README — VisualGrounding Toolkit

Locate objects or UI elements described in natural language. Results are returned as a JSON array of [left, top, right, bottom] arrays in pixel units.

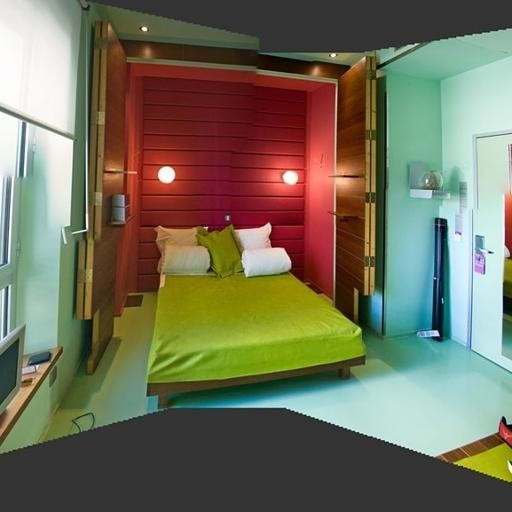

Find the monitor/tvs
[[0, 324, 25, 417]]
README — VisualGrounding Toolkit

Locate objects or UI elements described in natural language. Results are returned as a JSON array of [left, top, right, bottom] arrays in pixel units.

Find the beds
[[146, 254, 367, 409]]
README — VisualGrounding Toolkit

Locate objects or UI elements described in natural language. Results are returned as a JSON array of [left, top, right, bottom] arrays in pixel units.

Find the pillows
[[155, 223, 294, 277]]
